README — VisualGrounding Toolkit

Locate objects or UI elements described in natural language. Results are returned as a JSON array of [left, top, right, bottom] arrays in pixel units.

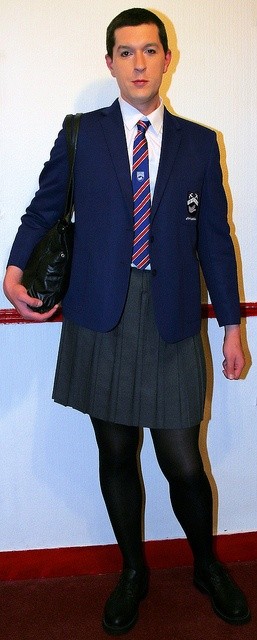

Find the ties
[[132, 120, 151, 271]]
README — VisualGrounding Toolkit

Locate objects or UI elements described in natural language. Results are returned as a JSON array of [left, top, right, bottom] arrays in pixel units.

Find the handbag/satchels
[[22, 211, 70, 314]]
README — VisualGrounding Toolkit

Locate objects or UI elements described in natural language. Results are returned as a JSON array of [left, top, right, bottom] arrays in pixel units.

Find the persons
[[3, 8, 252, 637]]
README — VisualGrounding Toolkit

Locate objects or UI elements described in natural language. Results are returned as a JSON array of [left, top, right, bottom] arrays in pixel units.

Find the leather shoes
[[193, 558, 250, 625], [102, 566, 149, 635]]
